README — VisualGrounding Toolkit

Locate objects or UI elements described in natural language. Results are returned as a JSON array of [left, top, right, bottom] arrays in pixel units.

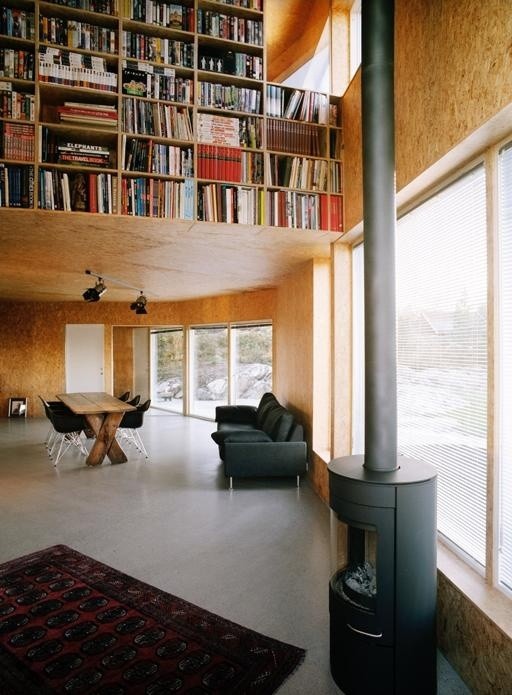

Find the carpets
[[2, 539, 309, 694]]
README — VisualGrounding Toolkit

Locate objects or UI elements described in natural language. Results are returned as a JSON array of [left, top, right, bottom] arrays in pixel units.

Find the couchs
[[214, 392, 310, 492]]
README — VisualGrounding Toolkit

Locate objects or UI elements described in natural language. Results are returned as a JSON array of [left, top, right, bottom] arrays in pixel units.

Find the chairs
[[34, 387, 154, 469]]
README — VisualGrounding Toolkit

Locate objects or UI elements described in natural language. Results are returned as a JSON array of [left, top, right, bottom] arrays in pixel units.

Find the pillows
[[222, 405, 260, 426], [208, 428, 275, 447]]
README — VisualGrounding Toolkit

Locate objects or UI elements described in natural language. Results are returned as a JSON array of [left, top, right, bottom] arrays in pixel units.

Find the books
[[196, 0, 263, 46], [1, 6, 35, 210], [197, 46, 264, 225], [39, 14, 120, 214], [266, 84, 343, 233], [47, 1, 196, 33], [121, 28, 195, 220]]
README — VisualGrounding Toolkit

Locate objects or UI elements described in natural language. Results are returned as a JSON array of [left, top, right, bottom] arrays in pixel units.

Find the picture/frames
[[8, 396, 29, 419]]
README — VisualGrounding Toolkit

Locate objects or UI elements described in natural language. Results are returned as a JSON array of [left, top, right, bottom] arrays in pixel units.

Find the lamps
[[82, 274, 150, 316]]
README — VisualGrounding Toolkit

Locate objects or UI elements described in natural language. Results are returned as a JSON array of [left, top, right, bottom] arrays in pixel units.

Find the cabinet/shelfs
[[0, 1, 346, 234], [325, 471, 440, 694]]
